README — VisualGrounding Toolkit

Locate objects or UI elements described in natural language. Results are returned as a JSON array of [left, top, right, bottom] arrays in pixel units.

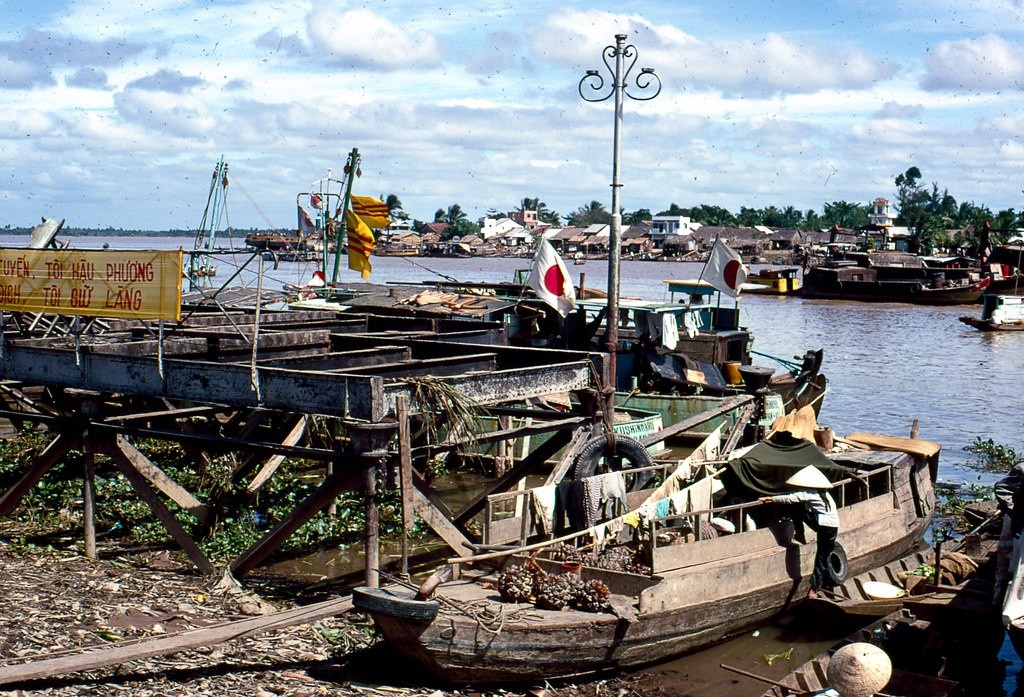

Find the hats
[[786, 464, 833, 489], [827, 641, 892, 697]]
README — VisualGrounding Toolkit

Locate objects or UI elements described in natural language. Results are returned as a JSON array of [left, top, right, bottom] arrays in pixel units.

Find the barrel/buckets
[[1001, 264, 1010, 276], [722, 360, 742, 384]]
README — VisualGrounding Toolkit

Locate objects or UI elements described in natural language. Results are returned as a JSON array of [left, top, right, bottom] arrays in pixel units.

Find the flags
[[526, 235, 577, 320], [310, 192, 328, 210], [347, 191, 392, 229], [701, 233, 749, 296], [297, 204, 317, 234], [341, 210, 376, 282]]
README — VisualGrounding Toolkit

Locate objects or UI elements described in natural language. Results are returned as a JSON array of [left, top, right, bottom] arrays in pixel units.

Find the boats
[[958, 294, 1024, 332], [804, 531, 999, 627], [351, 418, 947, 688], [761, 557, 1007, 697], [262, 251, 322, 262], [744, 243, 991, 306], [283, 269, 829, 474]]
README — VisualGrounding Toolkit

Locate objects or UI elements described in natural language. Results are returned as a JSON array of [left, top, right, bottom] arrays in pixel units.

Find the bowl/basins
[[863, 581, 905, 600]]
